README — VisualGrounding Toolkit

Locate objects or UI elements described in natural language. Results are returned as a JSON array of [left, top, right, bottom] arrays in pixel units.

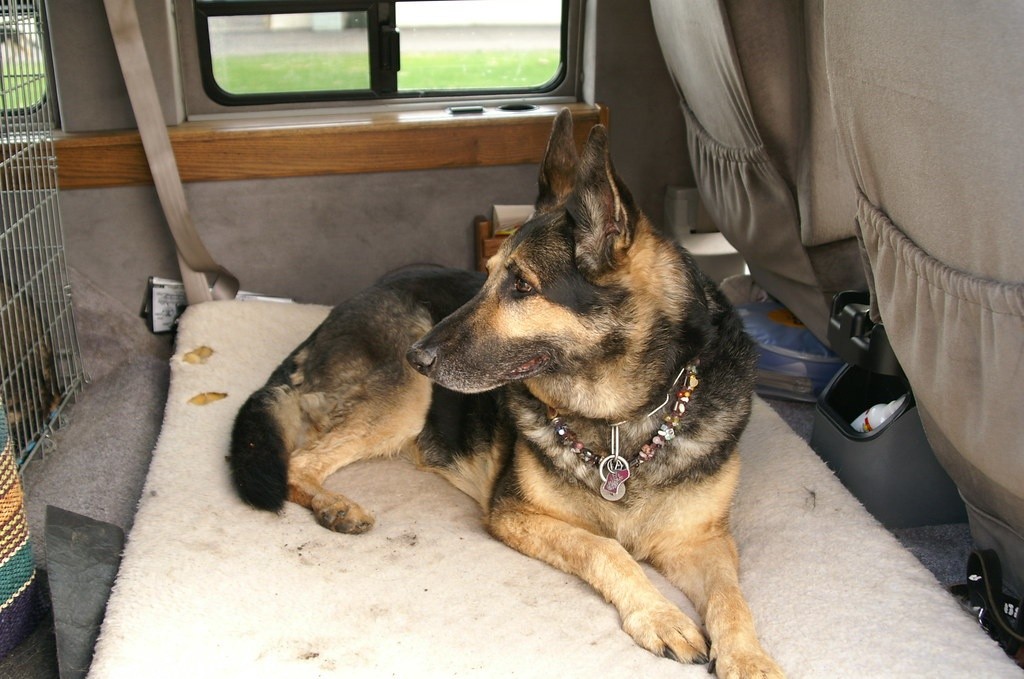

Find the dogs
[[230, 107, 786, 679]]
